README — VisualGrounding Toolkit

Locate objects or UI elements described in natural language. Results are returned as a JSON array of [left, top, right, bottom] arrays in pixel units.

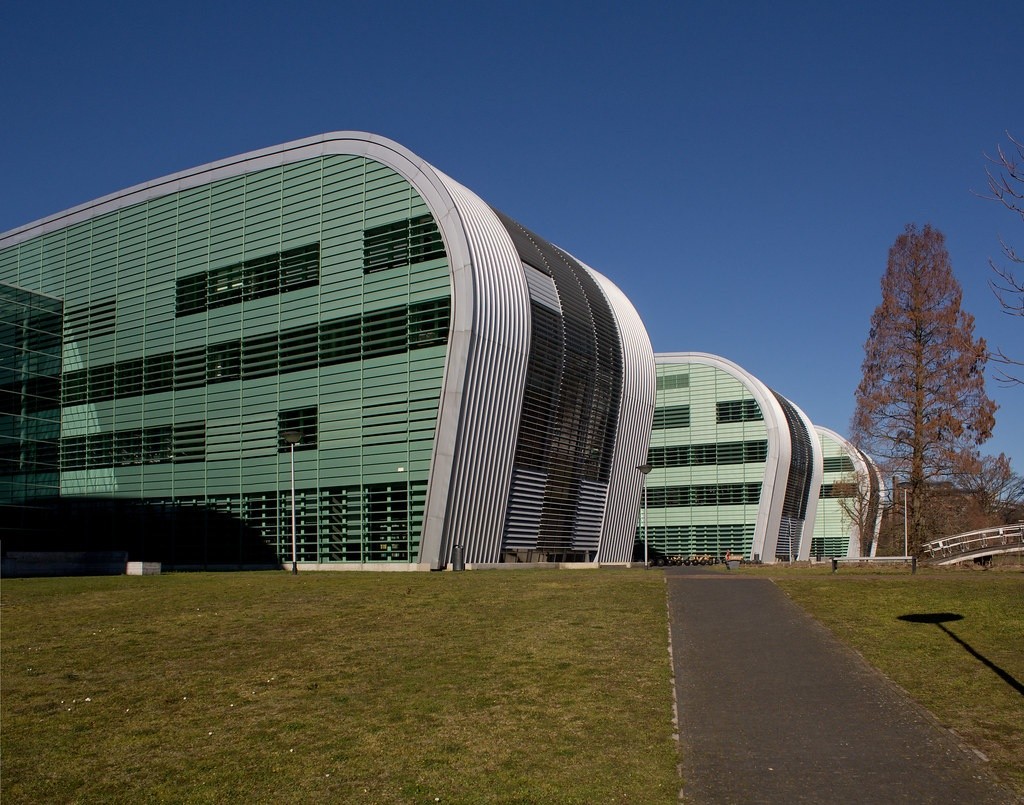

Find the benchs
[[126, 561, 161, 576]]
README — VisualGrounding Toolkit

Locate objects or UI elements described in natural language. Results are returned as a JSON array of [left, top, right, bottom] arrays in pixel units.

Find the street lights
[[636, 465, 655, 566], [787, 511, 795, 565], [280, 429, 306, 574], [897, 482, 912, 557]]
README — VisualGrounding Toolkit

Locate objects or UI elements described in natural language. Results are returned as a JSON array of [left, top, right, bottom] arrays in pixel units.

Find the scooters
[[665, 556, 726, 566]]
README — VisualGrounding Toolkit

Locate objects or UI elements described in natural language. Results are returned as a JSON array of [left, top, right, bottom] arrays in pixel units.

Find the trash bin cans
[[452, 544, 464, 572]]
